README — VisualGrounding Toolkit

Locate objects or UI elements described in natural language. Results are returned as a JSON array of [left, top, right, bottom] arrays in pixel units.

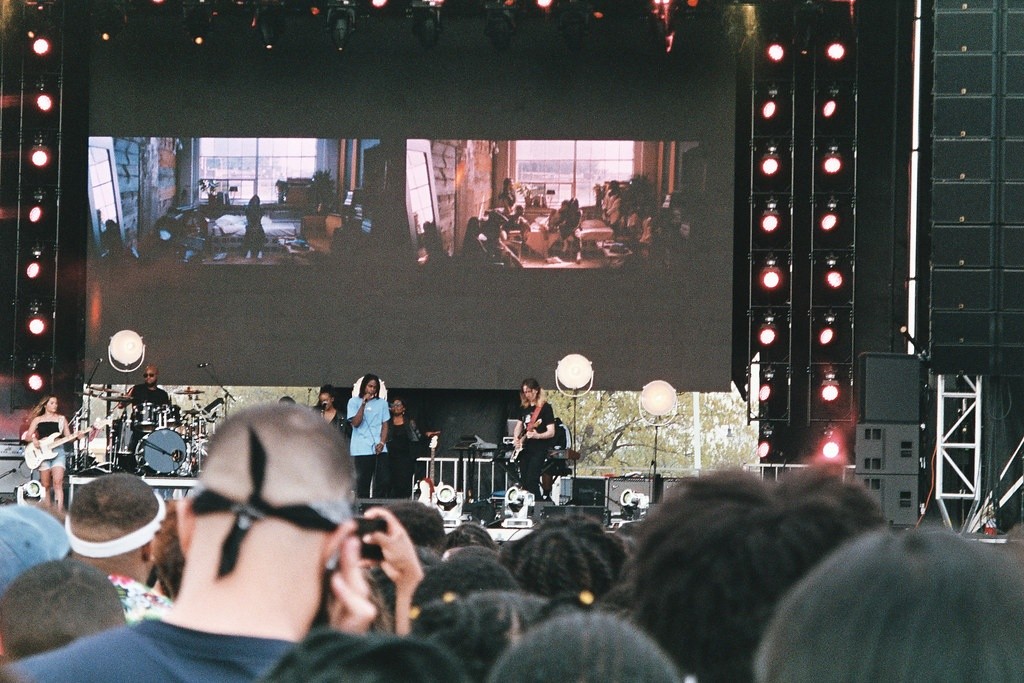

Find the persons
[[242, 195, 266, 258], [315, 384, 352, 439], [752, 529, 1024, 683], [0, 402, 680, 683], [25, 395, 83, 510], [118, 366, 169, 451], [385, 398, 441, 498], [595, 180, 651, 250], [617, 468, 886, 683], [463, 178, 531, 268], [513, 378, 555, 501], [347, 374, 391, 497], [538, 197, 583, 263]]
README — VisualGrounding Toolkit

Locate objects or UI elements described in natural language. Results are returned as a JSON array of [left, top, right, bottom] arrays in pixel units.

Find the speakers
[[607, 476, 693, 519], [0, 459, 41, 492], [540, 504, 607, 528], [853, 350, 921, 527], [572, 476, 607, 506]]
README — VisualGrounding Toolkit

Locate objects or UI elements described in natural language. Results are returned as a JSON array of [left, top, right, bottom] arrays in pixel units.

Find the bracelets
[[381, 442, 384, 445]]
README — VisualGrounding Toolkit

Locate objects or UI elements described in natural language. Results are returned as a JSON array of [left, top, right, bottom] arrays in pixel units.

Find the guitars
[[417, 435, 439, 504], [23, 415, 113, 470], [509, 418, 543, 463]]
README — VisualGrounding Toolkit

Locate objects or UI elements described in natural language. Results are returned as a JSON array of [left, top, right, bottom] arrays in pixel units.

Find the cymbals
[[173, 389, 205, 395], [73, 392, 102, 398], [86, 386, 120, 393], [101, 396, 142, 403]]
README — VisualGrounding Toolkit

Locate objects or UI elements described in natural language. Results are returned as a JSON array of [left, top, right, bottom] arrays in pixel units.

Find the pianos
[[481, 448, 581, 504]]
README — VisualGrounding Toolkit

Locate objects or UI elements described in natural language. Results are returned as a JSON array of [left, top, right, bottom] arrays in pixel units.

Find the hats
[[0, 504, 71, 592]]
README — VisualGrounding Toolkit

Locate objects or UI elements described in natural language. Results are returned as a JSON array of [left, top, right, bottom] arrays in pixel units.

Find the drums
[[132, 400, 157, 426], [134, 426, 187, 477], [150, 405, 179, 422], [106, 419, 141, 456]]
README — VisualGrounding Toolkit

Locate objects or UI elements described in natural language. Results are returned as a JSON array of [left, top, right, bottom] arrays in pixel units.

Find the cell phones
[[353, 517, 386, 560]]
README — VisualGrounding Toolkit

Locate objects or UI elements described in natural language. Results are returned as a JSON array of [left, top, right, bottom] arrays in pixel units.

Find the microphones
[[197, 362, 211, 367], [321, 401, 328, 412], [12, 469, 16, 473], [364, 399, 367, 404]]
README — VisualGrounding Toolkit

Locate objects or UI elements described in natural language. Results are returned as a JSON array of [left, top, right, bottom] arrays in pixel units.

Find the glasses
[[143, 372, 157, 378]]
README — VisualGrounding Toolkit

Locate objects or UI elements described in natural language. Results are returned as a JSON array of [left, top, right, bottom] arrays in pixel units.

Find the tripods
[[76, 408, 120, 477]]
